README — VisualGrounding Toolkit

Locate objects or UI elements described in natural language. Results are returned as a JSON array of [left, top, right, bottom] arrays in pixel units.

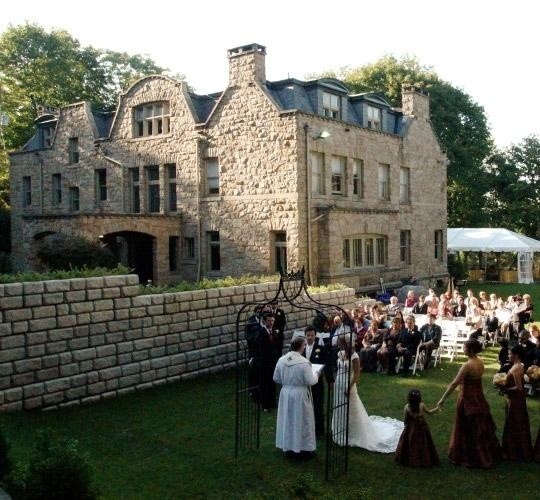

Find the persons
[[248, 313, 284, 413], [394, 389, 444, 468], [436, 338, 503, 470], [243, 288, 540, 377], [272, 336, 319, 462], [494, 345, 534, 463], [300, 326, 334, 439], [331, 333, 405, 454]]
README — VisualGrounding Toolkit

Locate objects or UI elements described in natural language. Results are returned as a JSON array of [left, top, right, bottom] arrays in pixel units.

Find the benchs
[[387, 314, 498, 376]]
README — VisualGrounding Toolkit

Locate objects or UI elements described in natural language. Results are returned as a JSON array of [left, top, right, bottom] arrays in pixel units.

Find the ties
[[269, 330, 272, 342]]
